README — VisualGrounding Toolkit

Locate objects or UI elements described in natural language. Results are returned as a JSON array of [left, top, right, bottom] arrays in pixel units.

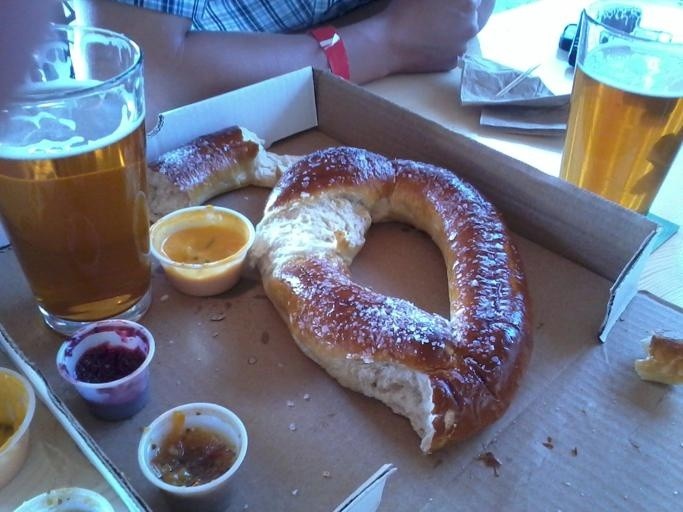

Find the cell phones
[[559, 24, 674, 53]]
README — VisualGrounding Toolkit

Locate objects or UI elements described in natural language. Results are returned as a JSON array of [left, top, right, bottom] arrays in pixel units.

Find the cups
[[0, 23, 152, 339], [0, 367, 36, 491], [150, 205, 256, 297], [14, 488, 115, 512], [138, 402, 247, 502], [57, 320, 156, 418], [556, 1, 682, 222]]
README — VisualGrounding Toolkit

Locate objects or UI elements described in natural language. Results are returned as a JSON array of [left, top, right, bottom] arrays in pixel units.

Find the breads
[[148, 124, 305, 223], [256, 147, 533, 455], [635, 329, 683, 385]]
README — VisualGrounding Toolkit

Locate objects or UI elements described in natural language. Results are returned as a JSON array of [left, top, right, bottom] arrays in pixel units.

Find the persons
[[1, 0, 495, 135]]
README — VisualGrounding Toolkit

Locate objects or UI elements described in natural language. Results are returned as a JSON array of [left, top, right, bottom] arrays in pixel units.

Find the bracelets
[[307, 24, 351, 81]]
